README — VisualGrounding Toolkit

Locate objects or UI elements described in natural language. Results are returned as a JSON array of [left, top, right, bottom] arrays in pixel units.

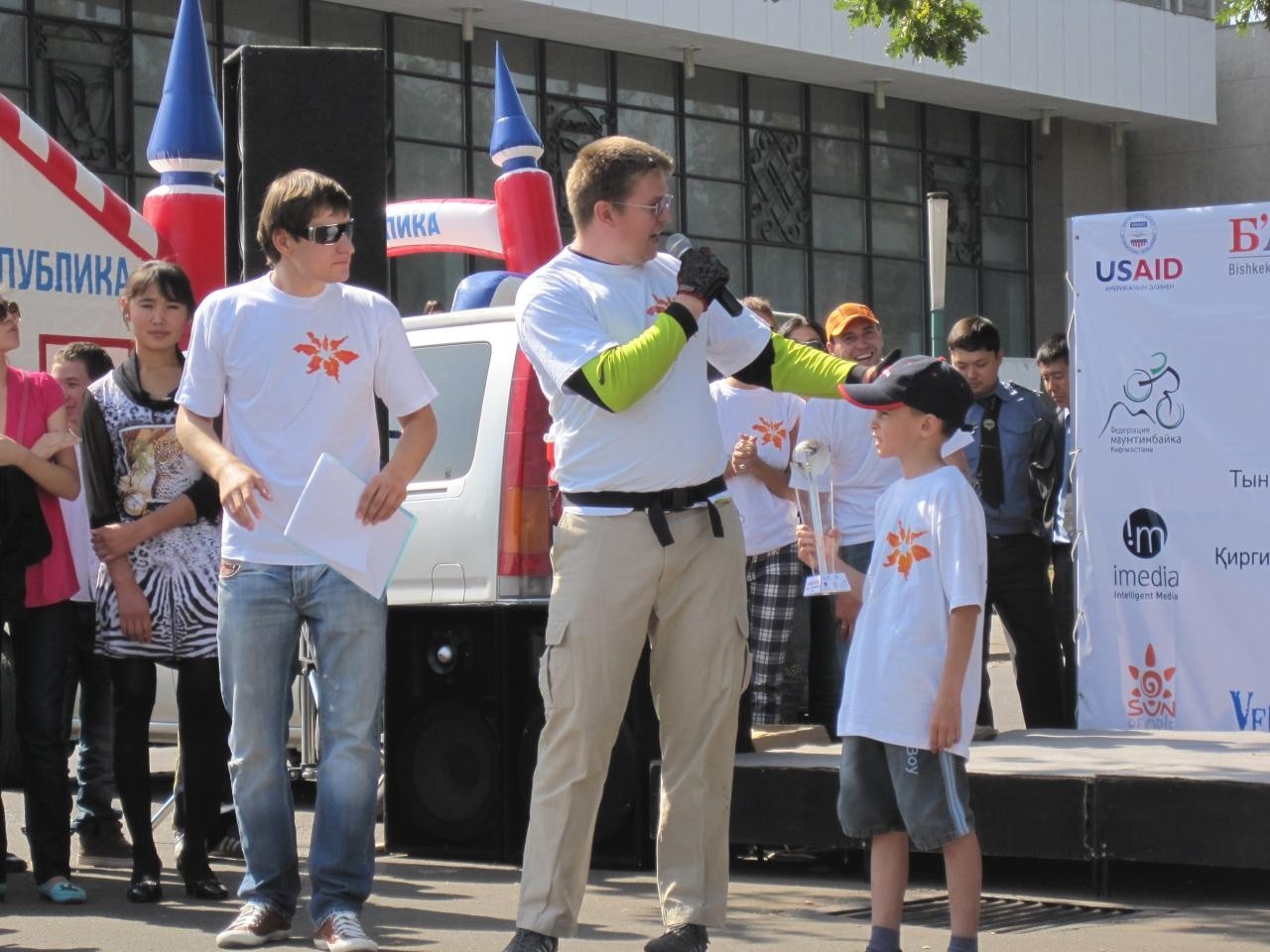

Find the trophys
[[793, 439, 852, 598]]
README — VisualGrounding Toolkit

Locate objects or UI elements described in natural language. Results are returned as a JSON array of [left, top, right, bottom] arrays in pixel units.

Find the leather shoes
[[35, 877, 88, 904]]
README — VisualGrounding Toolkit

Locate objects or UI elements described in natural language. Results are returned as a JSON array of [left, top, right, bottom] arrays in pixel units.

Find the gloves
[[675, 244, 730, 313], [867, 348, 902, 385]]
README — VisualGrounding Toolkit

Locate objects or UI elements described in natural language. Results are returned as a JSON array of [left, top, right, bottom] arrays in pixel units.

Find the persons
[[800, 357, 987, 952], [2, 261, 244, 904], [175, 170, 437, 951], [710, 299, 811, 727], [504, 137, 902, 952], [790, 304, 974, 717], [947, 314, 1077, 732], [779, 316, 829, 357]]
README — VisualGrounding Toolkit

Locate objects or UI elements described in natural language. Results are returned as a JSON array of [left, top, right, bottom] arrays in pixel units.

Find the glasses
[[0, 302, 20, 322], [294, 217, 355, 247], [613, 193, 675, 218], [794, 337, 826, 354]]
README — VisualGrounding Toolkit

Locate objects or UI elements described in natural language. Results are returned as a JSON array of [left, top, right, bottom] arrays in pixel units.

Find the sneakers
[[170, 826, 244, 860], [77, 836, 135, 868], [216, 900, 294, 947], [313, 910, 378, 952], [501, 928, 560, 952], [643, 923, 712, 952]]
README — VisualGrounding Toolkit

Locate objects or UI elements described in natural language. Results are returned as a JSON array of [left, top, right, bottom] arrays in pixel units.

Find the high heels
[[174, 851, 229, 900], [126, 857, 161, 904]]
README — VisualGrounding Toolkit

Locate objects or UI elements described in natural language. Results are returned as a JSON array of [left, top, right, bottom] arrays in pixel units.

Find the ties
[[976, 393, 1009, 512]]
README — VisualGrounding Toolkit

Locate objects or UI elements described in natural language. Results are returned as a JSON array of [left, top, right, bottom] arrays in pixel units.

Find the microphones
[[666, 233, 743, 317]]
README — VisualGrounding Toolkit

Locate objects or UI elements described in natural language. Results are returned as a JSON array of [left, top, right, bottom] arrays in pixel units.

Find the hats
[[824, 302, 880, 344], [837, 353, 972, 433]]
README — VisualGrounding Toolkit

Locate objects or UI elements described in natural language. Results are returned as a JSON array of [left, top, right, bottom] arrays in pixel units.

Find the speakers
[[385, 598, 660, 870], [218, 46, 391, 297]]
